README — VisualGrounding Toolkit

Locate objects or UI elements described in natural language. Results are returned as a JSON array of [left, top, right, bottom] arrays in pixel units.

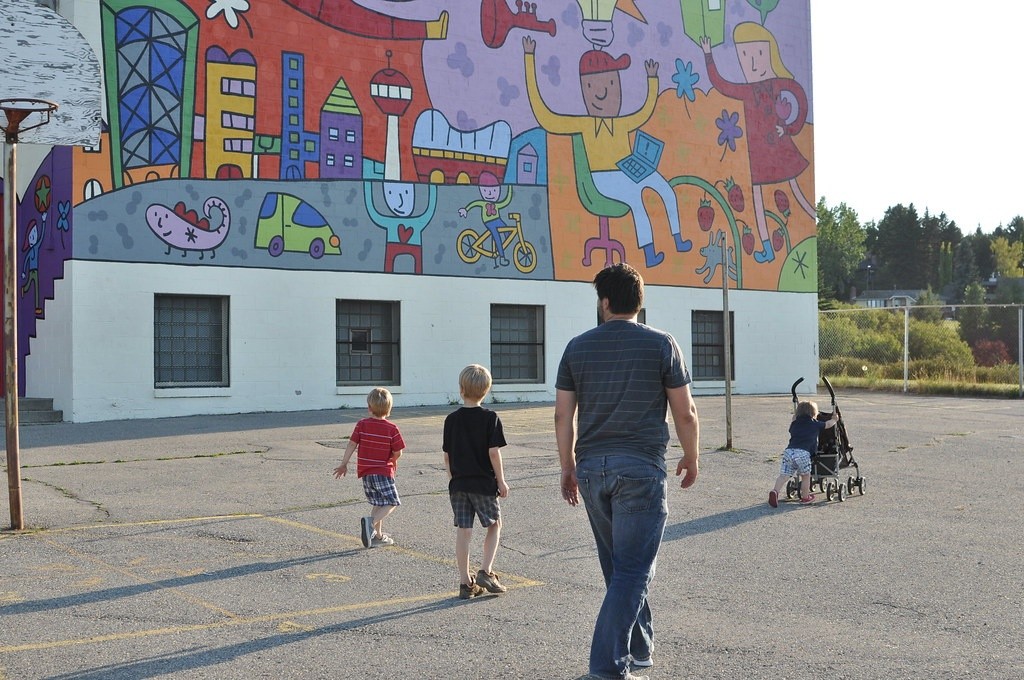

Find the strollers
[[785, 373, 867, 504]]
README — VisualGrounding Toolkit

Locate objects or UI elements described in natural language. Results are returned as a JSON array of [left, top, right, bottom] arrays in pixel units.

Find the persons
[[768, 401, 839, 508], [332, 388, 405, 549], [442, 364, 509, 600], [554, 263, 700, 680]]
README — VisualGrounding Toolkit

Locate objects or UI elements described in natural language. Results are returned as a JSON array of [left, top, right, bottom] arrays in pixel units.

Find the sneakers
[[459, 575, 486, 598], [371, 534, 393, 547], [476, 570, 506, 593], [361, 517, 377, 548]]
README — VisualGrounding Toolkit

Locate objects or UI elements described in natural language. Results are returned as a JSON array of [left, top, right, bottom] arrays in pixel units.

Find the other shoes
[[800, 495, 816, 504], [632, 656, 653, 666], [627, 673, 649, 680], [768, 489, 778, 507]]
